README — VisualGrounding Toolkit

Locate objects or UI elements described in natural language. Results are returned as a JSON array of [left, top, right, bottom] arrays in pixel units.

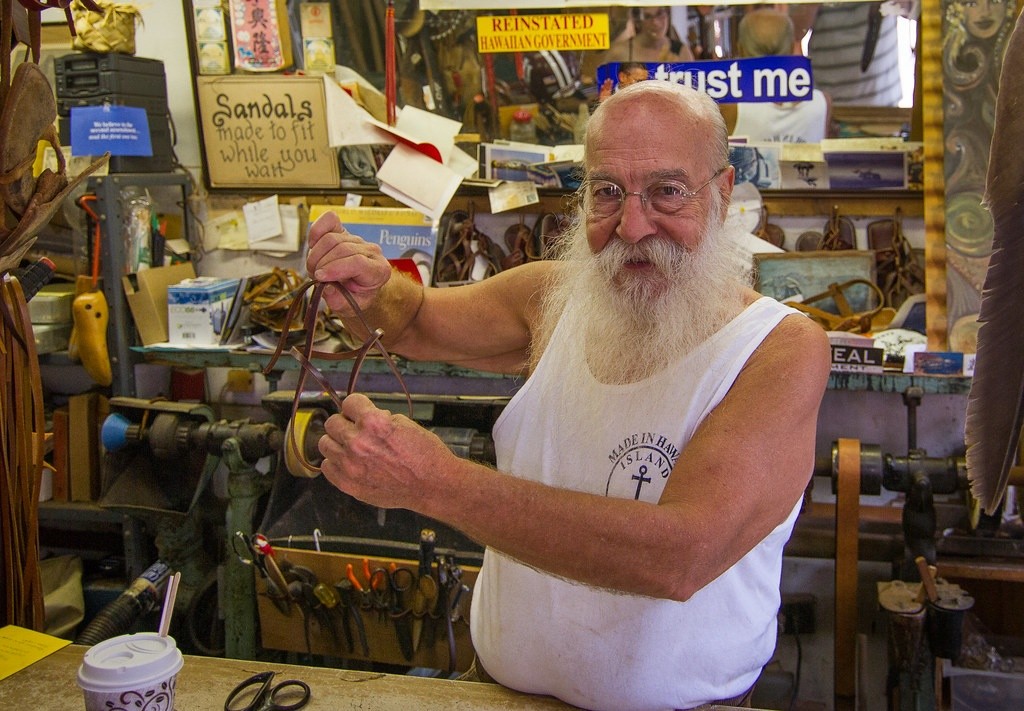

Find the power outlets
[[783, 596, 816, 633]]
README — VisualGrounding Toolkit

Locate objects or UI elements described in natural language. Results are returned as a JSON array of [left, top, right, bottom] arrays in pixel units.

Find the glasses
[[578, 171, 721, 217], [643, 9, 667, 25]]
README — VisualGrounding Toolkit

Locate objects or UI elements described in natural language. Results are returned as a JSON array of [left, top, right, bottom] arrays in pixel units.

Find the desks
[[0, 643, 750, 710]]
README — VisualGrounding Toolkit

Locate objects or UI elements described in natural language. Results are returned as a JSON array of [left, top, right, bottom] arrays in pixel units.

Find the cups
[[77, 632, 184, 711]]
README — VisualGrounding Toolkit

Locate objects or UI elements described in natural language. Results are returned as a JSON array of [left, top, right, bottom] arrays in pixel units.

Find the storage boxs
[[166, 276, 247, 346], [26, 283, 77, 355], [53, 47, 174, 173], [121, 263, 196, 344]]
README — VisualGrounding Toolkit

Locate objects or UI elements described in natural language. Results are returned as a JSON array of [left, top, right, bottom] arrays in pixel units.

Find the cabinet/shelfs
[[17, 168, 198, 635]]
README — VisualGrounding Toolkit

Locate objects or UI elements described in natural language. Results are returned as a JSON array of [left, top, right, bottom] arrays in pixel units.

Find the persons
[[720, 11, 830, 142], [587, 7, 696, 80], [306, 79, 830, 711]]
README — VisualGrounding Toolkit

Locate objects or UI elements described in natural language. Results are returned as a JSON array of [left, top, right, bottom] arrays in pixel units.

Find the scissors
[[411, 528, 449, 623], [224, 670, 311, 711], [369, 568, 414, 664]]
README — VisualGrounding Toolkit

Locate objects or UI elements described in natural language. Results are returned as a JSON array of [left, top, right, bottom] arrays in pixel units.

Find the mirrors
[[179, 0, 924, 200]]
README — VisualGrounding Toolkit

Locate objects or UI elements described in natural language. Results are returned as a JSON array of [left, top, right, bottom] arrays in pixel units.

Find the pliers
[[233, 532, 397, 659], [418, 558, 470, 672]]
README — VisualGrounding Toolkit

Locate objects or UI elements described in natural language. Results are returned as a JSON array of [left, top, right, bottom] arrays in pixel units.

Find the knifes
[[413, 529, 437, 653]]
